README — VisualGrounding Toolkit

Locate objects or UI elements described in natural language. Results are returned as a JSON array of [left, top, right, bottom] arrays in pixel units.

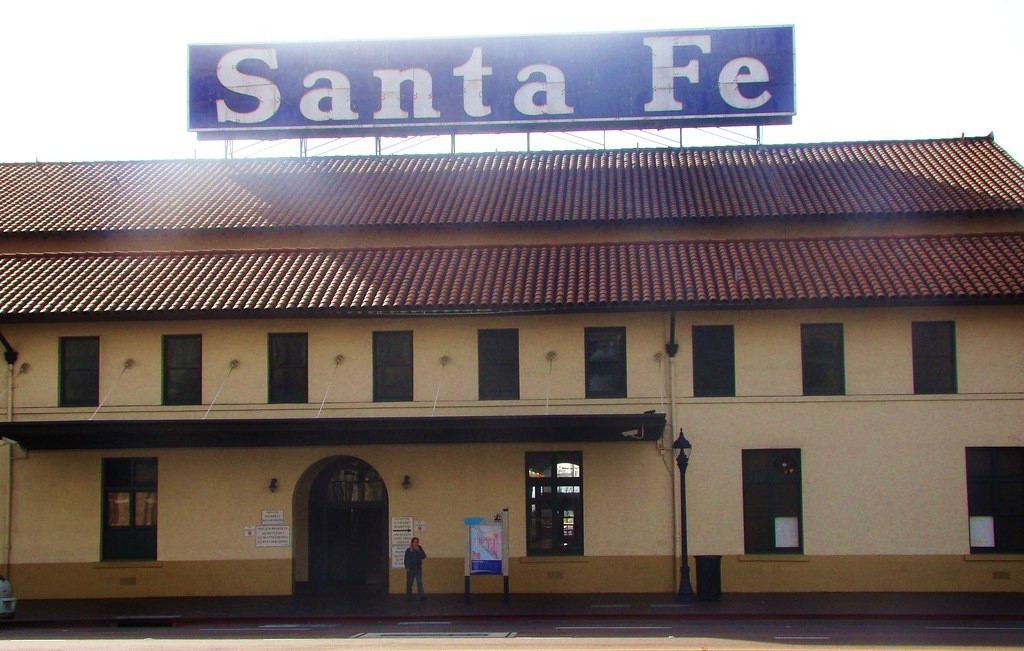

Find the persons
[[403, 537, 429, 601]]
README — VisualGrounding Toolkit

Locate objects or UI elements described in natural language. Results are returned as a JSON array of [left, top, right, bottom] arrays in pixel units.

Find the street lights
[[672, 427, 697, 606]]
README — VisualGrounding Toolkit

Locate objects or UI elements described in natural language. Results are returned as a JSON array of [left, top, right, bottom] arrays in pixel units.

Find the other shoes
[[407, 598, 412, 601], [420, 596, 427, 601]]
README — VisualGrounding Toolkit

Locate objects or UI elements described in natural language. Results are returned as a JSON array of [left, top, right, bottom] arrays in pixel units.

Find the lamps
[[402, 475, 411, 490], [268, 478, 278, 492]]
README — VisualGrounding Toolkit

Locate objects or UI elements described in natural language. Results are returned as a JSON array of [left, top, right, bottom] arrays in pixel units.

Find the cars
[[0, 573, 18, 622]]
